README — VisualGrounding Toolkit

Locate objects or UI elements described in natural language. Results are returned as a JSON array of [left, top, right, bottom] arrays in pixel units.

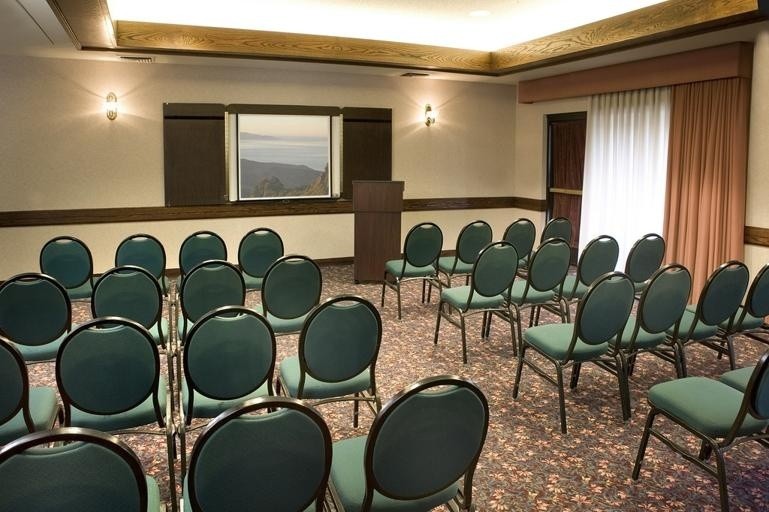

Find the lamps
[[107, 93, 117, 119], [426, 103, 434, 126]]
